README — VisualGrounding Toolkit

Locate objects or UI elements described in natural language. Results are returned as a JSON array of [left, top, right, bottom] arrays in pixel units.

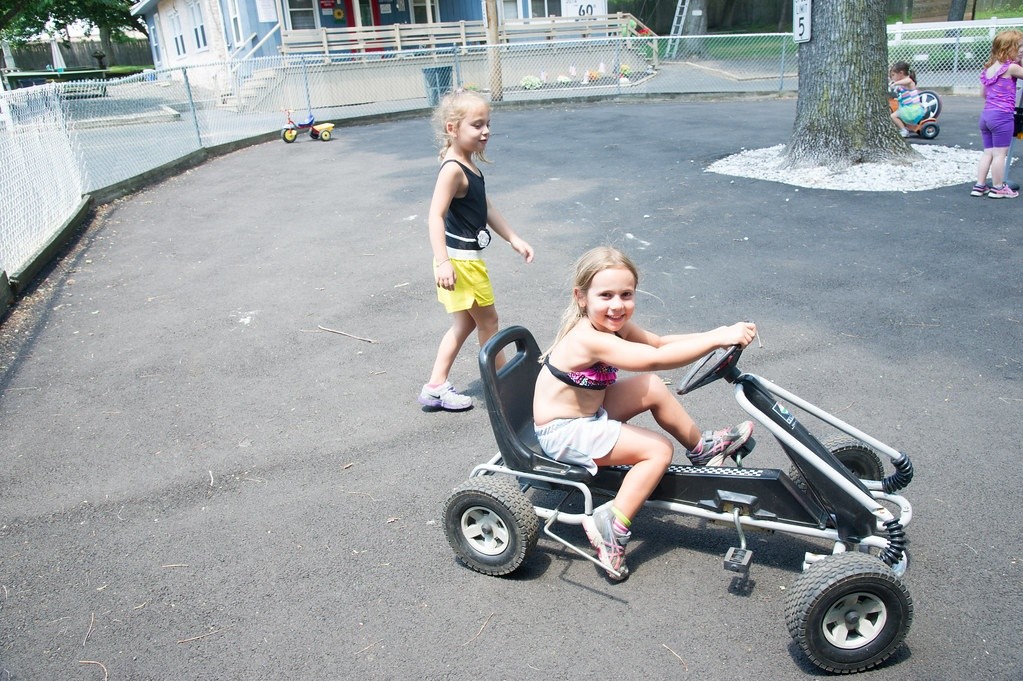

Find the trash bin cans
[[422, 65, 453, 107], [143, 69, 155, 81]]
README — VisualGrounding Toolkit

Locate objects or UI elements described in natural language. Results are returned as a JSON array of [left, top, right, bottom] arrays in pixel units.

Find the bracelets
[[437, 258, 450, 267]]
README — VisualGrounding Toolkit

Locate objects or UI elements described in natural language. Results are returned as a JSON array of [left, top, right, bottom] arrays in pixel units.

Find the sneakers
[[419, 378, 473, 409], [581, 508, 631, 580], [685, 421, 753, 467], [970, 184, 991, 196], [899, 130, 910, 138], [988, 182, 1019, 198]]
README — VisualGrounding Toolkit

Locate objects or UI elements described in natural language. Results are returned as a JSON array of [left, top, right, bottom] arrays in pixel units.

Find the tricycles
[[885, 83, 939, 140], [282, 108, 334, 143]]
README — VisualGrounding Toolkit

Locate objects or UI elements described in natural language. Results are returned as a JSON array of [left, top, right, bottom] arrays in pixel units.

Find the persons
[[889, 62, 925, 138], [532, 245, 756, 581], [970, 30, 1023, 198], [418, 86, 534, 411]]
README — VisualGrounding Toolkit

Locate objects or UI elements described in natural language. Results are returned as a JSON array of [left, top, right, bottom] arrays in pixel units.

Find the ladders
[[665, 0, 690, 60]]
